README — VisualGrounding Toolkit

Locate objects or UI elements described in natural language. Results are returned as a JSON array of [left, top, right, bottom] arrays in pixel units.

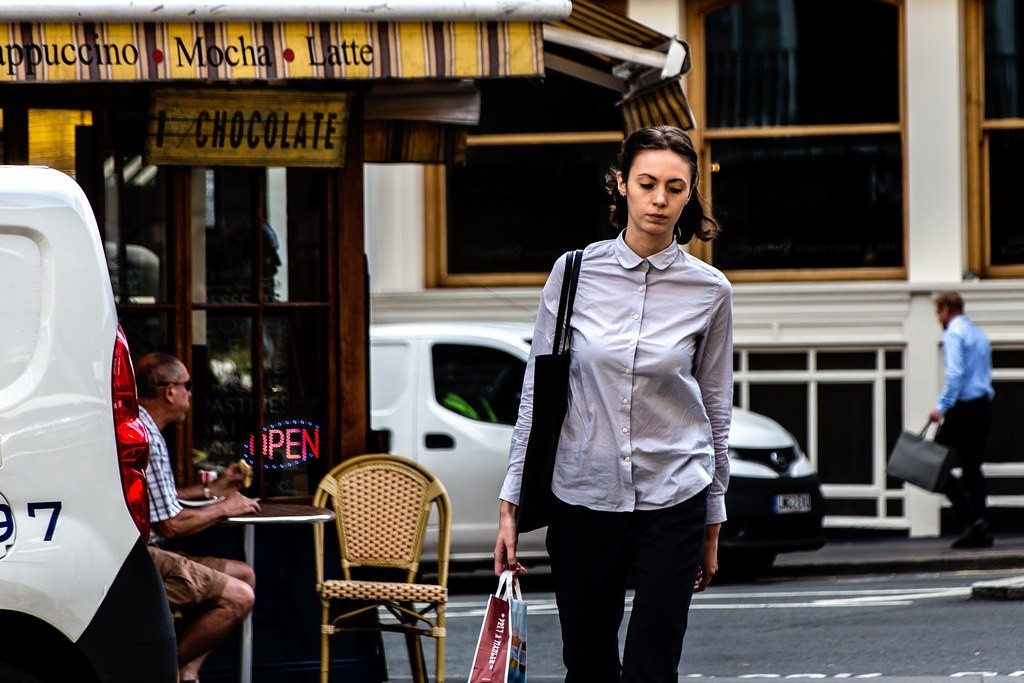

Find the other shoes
[[952, 517, 986, 550], [962, 535, 994, 550]]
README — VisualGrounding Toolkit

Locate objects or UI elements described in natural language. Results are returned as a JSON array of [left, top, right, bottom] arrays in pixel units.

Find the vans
[[368, 320, 828, 588]]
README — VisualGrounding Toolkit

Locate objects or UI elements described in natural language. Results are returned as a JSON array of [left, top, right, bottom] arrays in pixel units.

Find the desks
[[219, 504, 335, 683]]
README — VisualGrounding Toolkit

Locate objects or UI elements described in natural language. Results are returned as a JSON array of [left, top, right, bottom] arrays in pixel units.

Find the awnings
[[0, 0, 699, 165]]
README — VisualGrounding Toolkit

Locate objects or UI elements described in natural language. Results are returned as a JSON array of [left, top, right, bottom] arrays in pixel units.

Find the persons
[[928, 291, 1000, 549], [133, 351, 255, 683], [496, 124, 734, 683], [442, 349, 495, 424]]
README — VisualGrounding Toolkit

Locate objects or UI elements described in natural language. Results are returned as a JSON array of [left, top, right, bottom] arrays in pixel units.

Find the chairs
[[311, 453, 452, 683]]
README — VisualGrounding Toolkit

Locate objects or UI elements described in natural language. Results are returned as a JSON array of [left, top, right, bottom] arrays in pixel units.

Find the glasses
[[157, 377, 191, 391]]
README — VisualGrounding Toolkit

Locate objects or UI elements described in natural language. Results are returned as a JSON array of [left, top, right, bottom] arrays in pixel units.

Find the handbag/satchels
[[467, 571, 528, 683], [513, 250, 584, 534], [885, 418, 951, 492]]
